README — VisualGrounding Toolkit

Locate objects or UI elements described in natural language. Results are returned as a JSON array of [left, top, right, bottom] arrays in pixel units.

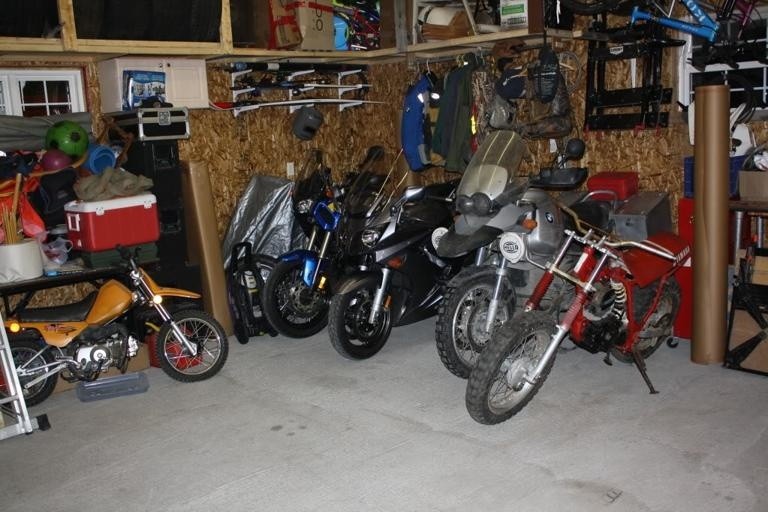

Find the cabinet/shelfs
[[0, 0, 609, 115]]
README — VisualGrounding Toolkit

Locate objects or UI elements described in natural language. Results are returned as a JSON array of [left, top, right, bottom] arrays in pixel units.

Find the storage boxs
[[64, 191, 161, 252], [571, 154, 751, 349]]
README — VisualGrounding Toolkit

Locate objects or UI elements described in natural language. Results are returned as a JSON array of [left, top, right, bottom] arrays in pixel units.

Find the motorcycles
[[0, 244, 230, 410]]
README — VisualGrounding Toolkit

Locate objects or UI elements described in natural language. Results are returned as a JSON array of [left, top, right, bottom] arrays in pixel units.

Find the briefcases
[[614, 191, 672, 240], [105, 106, 191, 142], [587, 171, 639, 201]]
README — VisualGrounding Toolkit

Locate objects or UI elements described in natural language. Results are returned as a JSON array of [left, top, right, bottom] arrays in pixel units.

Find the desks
[[0, 259, 162, 318]]
